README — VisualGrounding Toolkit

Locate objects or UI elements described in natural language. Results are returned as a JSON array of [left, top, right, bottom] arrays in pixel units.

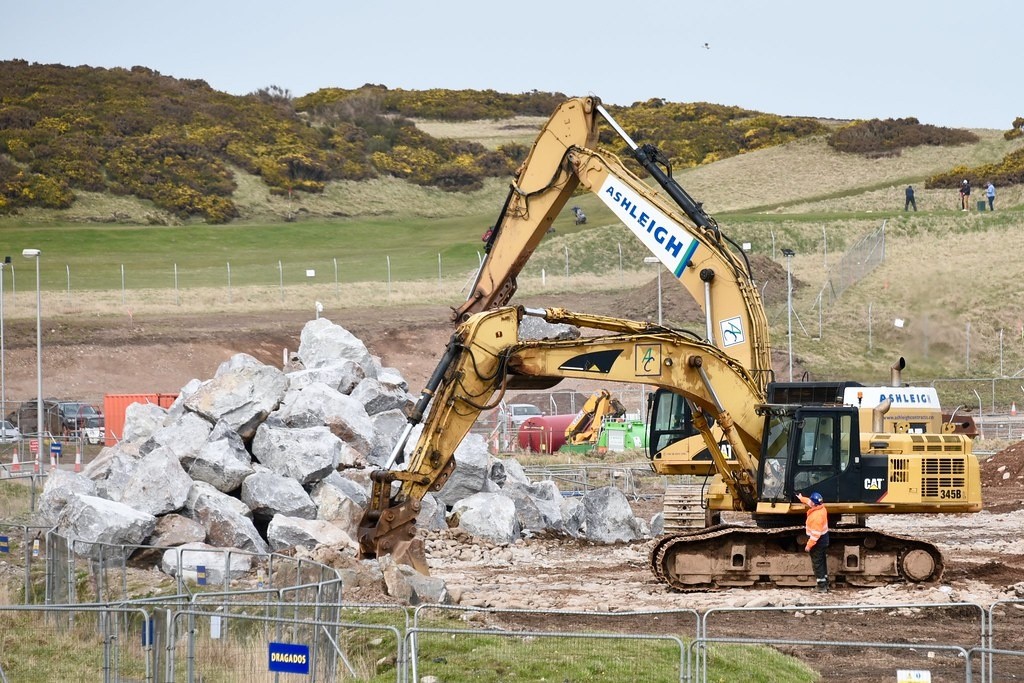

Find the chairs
[[814, 434, 833, 464]]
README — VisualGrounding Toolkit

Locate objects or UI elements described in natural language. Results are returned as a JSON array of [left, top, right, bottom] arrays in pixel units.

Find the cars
[[0, 420, 22, 444], [47, 402, 105, 436]]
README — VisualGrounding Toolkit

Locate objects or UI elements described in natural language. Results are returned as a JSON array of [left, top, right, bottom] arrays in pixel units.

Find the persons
[[796, 493, 831, 588], [905, 186, 917, 212], [960, 180, 970, 212], [982, 180, 995, 211]]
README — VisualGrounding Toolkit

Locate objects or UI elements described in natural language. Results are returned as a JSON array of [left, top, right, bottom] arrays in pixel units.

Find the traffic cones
[[33, 450, 39, 474], [10, 449, 21, 473], [1009, 401, 1016, 416], [50, 453, 57, 469], [73, 448, 81, 473]]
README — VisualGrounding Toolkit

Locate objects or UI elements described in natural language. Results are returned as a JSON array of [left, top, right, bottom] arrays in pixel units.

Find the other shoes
[[811, 588, 828, 593]]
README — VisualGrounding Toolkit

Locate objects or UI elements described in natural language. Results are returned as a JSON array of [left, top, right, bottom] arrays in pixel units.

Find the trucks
[[497, 403, 546, 433]]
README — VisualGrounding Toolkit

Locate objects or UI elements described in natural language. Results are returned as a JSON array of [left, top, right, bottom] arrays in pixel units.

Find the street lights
[[780, 248, 794, 383], [21, 248, 45, 483]]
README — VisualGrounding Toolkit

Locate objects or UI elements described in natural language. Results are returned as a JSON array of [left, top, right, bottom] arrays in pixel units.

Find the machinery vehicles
[[557, 387, 649, 453], [354, 93, 983, 593]]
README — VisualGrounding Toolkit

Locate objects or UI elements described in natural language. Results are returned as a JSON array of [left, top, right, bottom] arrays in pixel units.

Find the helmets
[[810, 492, 823, 505]]
